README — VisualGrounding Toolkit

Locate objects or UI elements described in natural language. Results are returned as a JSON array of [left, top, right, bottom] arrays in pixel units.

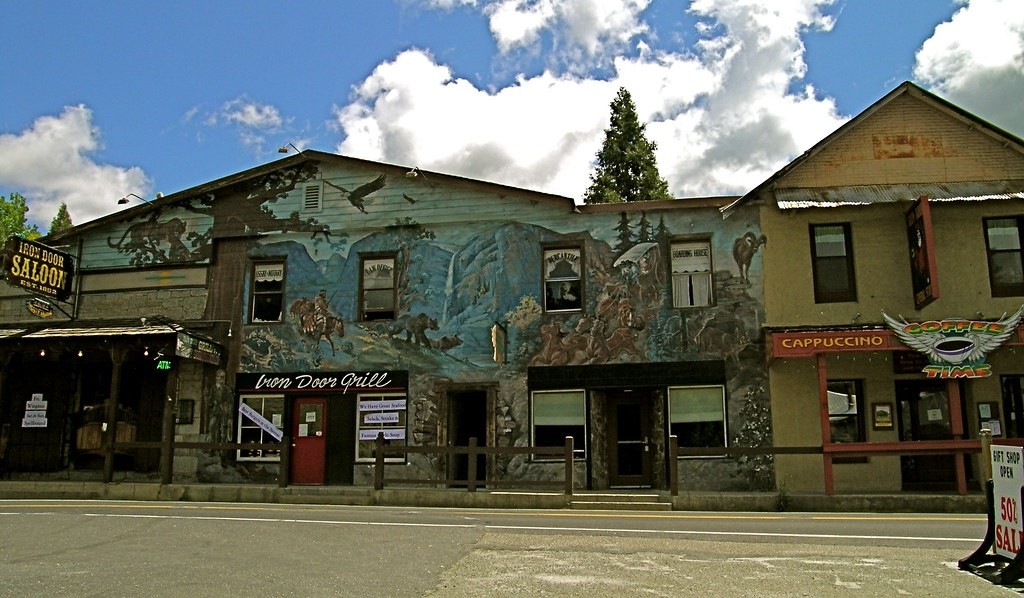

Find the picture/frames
[[872, 402, 894, 432]]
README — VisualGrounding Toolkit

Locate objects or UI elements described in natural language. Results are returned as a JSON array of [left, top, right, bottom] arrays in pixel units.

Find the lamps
[[405, 166, 433, 189], [278, 142, 306, 159], [118, 194, 153, 207]]
